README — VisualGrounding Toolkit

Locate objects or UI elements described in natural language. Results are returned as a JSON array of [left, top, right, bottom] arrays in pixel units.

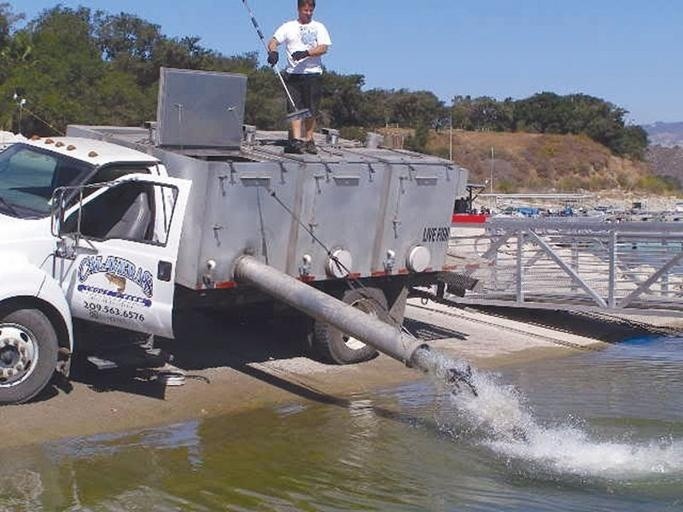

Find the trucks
[[0, 66, 464, 409]]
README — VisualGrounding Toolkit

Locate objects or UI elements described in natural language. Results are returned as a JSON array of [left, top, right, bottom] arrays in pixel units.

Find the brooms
[[240, 0, 312, 122]]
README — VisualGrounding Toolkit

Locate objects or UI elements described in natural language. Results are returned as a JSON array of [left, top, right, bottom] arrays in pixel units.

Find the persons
[[454, 196, 489, 217], [266, 1, 333, 155]]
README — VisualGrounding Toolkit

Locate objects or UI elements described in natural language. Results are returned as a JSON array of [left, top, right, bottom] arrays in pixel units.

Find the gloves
[[291, 50, 310, 61], [267, 51, 278, 68]]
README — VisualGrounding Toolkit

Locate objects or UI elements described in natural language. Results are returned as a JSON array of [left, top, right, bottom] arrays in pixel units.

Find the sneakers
[[305, 138, 317, 155], [284, 138, 303, 153]]
